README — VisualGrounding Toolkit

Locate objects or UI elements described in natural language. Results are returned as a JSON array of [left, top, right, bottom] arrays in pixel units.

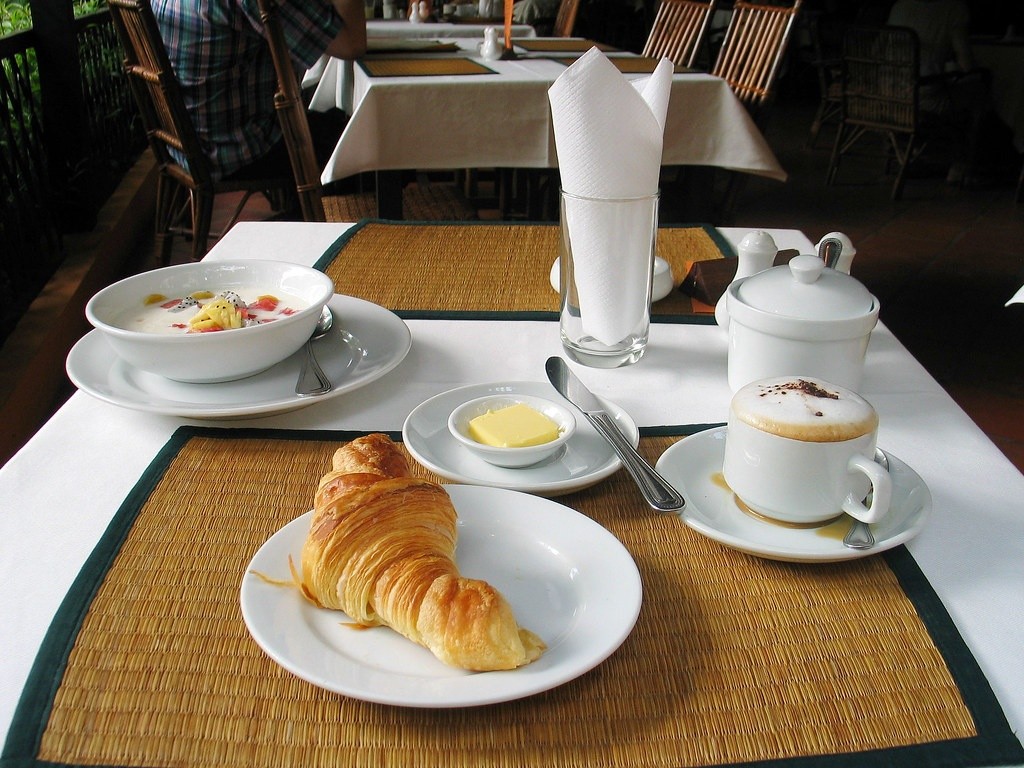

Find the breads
[[288, 431, 549, 671]]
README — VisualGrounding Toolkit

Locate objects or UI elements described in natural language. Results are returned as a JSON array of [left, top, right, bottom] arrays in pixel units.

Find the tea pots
[[479, 0, 492, 19]]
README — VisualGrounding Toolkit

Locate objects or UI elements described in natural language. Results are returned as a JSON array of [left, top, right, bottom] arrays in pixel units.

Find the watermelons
[[200, 298, 300, 331]]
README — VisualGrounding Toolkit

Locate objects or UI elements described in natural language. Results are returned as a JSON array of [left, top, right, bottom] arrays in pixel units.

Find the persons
[[871, 0, 1013, 178], [150, 0, 367, 221]]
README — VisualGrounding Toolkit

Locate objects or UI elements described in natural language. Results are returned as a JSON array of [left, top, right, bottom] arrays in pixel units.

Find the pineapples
[[191, 300, 243, 331]]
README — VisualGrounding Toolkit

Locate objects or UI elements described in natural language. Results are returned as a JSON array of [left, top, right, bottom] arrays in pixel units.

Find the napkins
[[548, 45, 675, 344]]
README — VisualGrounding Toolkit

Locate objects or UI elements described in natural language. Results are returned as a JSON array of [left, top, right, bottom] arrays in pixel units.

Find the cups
[[456, 6, 467, 17], [365, 0, 375, 20], [443, 4, 452, 14], [559, 184, 661, 370], [721, 403, 892, 523], [383, 5, 394, 20], [726, 254, 881, 395]]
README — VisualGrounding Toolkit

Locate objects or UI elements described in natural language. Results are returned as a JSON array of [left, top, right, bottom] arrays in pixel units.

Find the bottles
[[715, 230, 778, 333], [409, 2, 429, 24], [813, 232, 858, 275], [479, 28, 503, 60]]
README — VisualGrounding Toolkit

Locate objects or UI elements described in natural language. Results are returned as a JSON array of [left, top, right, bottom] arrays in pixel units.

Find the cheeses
[[468, 402, 559, 448]]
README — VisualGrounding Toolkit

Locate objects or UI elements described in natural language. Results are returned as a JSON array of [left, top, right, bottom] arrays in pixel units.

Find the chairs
[[644, 0, 714, 73], [111, 1, 295, 263], [805, 15, 912, 156], [551, 0, 577, 38], [715, 0, 802, 108], [254, 0, 477, 221], [822, 26, 978, 202]]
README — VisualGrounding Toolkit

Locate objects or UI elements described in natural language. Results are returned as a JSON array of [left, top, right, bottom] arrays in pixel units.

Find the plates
[[240, 484, 642, 708], [64, 293, 413, 421], [655, 426, 932, 563], [403, 381, 640, 498]]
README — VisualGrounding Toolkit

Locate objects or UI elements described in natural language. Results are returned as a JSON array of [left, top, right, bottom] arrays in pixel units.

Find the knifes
[[545, 356, 688, 515]]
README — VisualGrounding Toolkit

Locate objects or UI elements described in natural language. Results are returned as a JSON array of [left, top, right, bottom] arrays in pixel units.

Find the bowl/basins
[[85, 259, 335, 385], [446, 394, 577, 469]]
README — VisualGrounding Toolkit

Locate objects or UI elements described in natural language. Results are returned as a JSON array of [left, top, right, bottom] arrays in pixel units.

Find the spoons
[[842, 446, 890, 549], [294, 305, 334, 398]]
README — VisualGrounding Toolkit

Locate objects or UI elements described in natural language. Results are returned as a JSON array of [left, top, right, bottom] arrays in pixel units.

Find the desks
[[0, 222, 1024, 768], [298, 17, 786, 220]]
